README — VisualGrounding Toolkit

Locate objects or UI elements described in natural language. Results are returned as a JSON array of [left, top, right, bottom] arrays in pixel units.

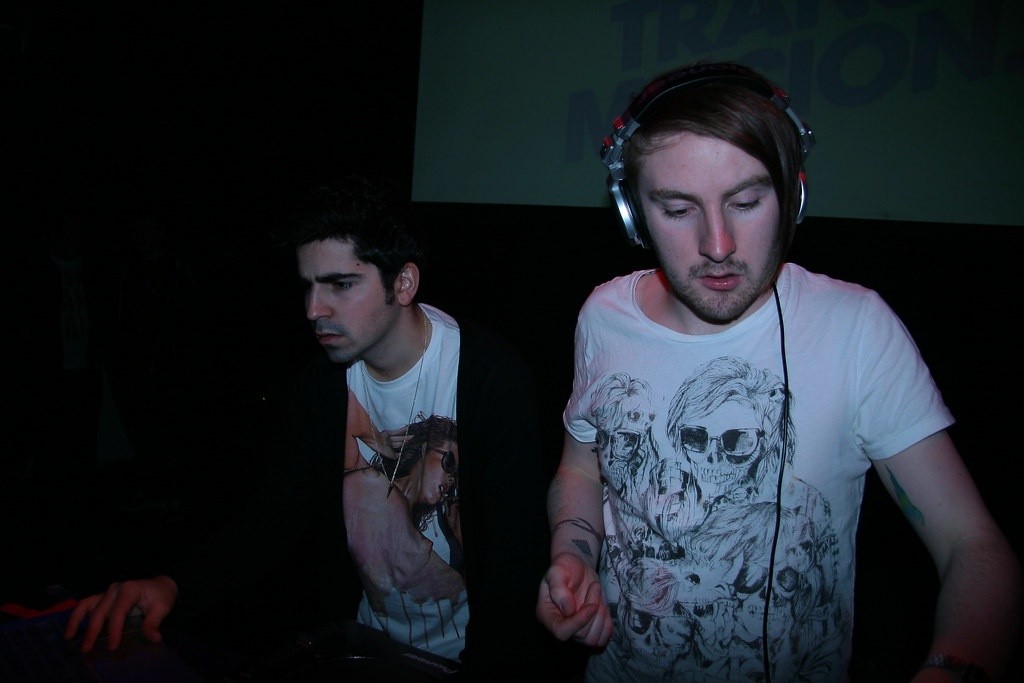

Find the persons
[[534, 62, 1024, 683], [35, 208, 582, 683]]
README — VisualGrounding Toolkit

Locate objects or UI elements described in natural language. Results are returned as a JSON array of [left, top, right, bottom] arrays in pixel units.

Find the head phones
[[599, 63, 816, 246]]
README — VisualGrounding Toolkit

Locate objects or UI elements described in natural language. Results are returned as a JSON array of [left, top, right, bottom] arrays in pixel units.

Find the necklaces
[[361, 320, 427, 495]]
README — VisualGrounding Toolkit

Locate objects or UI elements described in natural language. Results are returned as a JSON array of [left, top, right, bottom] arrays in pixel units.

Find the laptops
[[0, 612, 202, 683]]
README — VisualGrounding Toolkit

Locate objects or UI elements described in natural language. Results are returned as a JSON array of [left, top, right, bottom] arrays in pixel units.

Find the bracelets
[[924, 655, 989, 683]]
